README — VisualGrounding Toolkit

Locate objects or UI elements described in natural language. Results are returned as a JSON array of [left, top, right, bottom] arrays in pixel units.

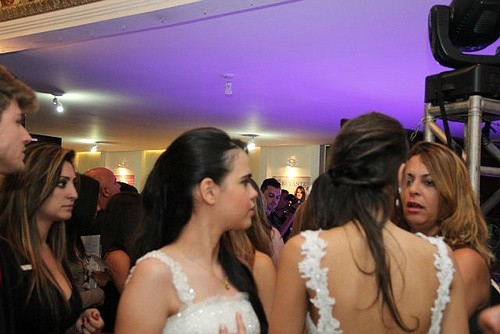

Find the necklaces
[[175, 240, 230, 291]]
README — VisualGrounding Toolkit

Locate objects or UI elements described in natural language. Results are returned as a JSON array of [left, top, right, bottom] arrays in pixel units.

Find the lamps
[[242, 134, 259, 151], [52, 96, 64, 113]]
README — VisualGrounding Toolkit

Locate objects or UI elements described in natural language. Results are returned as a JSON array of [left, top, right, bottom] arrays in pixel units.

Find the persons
[[0, 64, 500, 334]]
